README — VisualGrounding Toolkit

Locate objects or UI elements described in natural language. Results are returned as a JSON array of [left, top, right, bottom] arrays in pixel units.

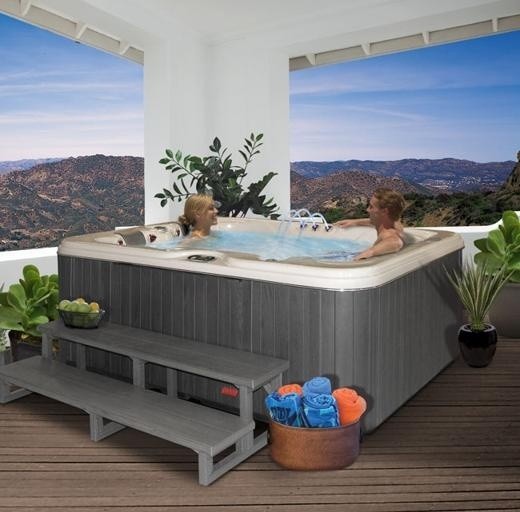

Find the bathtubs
[[56, 220, 465, 291]]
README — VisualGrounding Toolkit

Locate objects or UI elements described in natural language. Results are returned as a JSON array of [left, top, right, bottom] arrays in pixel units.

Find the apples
[[59, 297, 100, 326]]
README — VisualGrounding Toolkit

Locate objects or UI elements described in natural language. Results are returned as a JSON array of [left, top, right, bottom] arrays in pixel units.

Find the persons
[[173, 193, 222, 250], [331, 187, 409, 261]]
